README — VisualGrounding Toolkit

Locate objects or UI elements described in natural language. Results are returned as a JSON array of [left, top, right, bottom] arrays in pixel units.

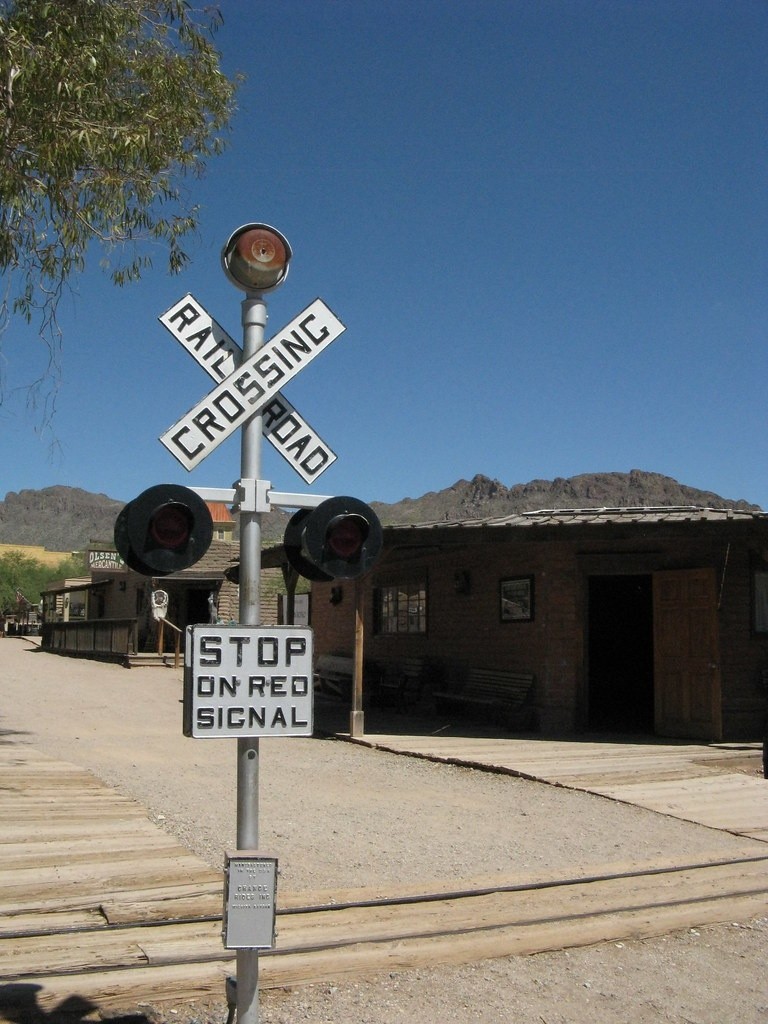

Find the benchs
[[431, 667, 534, 726], [314, 654, 353, 702], [365, 655, 426, 712]]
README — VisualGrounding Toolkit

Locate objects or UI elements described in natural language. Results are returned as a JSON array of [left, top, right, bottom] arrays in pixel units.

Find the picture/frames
[[749, 567, 768, 640], [498, 573, 535, 623]]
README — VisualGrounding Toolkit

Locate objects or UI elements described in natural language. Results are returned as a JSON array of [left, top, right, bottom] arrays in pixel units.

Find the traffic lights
[[306, 496, 383, 579], [127, 486, 214, 572]]
